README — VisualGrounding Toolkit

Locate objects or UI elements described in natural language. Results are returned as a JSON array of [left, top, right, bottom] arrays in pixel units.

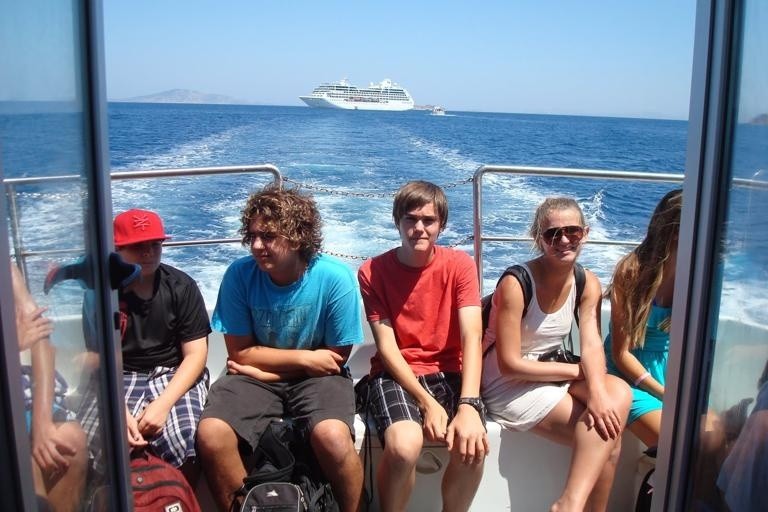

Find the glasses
[[542, 226, 583, 244]]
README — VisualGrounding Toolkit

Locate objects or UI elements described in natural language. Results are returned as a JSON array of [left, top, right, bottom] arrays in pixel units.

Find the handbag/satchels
[[538, 350, 580, 386]]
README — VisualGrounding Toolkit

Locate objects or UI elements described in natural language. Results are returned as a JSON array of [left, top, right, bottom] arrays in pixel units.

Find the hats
[[113, 208, 172, 247]]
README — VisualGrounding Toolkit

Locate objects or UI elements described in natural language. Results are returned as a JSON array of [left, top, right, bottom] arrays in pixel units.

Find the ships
[[299, 78, 413, 111]]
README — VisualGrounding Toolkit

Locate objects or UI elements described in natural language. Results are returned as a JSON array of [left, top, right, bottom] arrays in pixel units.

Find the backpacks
[[230, 419, 337, 512], [92, 444, 202, 512]]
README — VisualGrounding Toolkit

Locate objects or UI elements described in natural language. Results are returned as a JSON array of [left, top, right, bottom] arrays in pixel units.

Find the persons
[[77, 209, 213, 493], [194, 182, 366, 512], [602, 189, 727, 512], [9, 264, 87, 511], [481, 199, 633, 512], [357, 180, 489, 512], [715, 361, 768, 511]]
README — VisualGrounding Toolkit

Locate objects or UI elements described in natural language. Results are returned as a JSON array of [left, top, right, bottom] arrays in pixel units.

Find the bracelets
[[635, 372, 651, 387], [458, 397, 482, 412]]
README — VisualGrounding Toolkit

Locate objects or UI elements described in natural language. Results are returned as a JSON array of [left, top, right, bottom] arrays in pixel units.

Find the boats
[[432, 107, 445, 115]]
